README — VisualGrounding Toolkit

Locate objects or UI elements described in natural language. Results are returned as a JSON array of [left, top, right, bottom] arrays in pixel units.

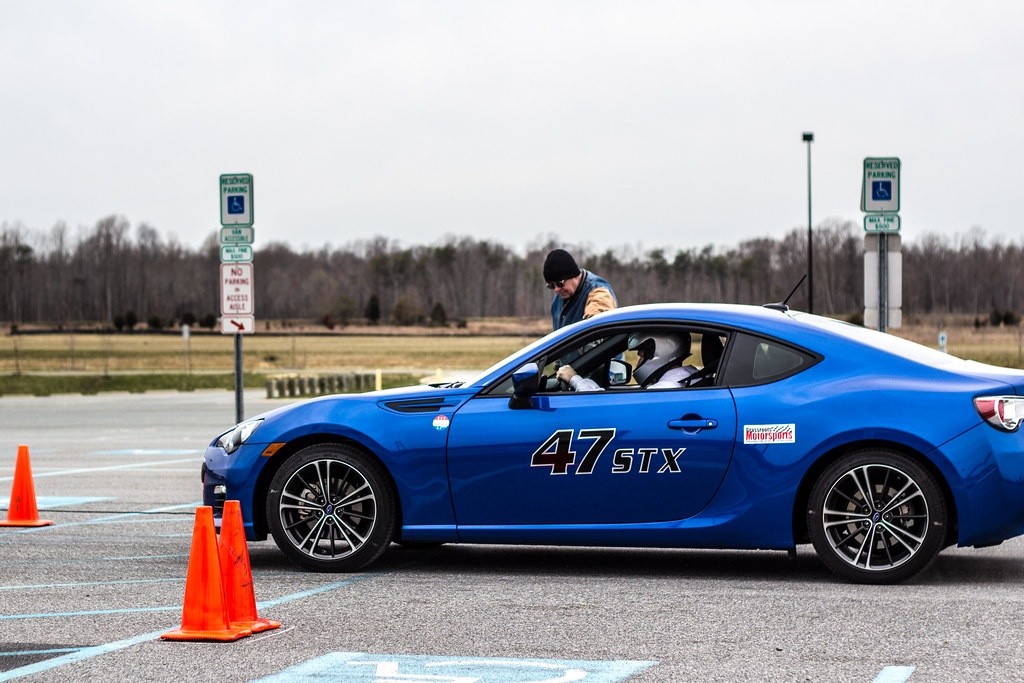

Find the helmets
[[627, 331, 691, 386]]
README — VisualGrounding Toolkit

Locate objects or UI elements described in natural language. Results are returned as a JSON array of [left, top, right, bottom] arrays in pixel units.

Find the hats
[[543, 249, 580, 283]]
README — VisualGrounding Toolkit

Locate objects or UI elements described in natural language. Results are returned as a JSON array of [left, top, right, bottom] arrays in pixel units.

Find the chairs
[[677, 334, 725, 388]]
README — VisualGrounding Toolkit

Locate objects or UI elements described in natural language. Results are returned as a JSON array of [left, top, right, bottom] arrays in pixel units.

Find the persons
[[542, 249, 625, 379], [556, 330, 703, 392]]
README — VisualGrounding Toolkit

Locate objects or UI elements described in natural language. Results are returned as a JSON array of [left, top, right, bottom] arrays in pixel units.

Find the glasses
[[546, 280, 565, 289]]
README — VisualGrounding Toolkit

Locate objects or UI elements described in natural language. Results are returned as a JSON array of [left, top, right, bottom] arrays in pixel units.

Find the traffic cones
[[0, 444, 54, 527], [160, 505, 255, 643], [218, 499, 282, 634]]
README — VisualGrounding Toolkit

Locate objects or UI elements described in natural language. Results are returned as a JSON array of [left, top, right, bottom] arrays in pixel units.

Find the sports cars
[[192, 302, 1024, 587]]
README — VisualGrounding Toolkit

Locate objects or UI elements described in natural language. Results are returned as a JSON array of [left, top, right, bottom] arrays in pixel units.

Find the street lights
[[801, 132, 814, 315]]
